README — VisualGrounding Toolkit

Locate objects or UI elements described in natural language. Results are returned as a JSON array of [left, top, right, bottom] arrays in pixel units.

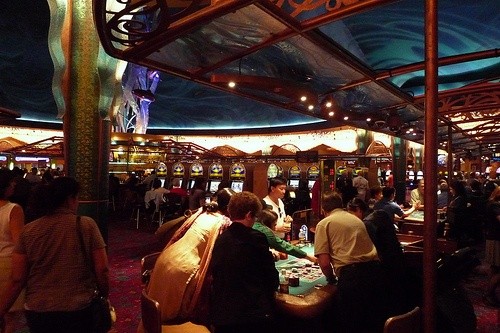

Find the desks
[[272, 239, 333, 321], [394, 210, 447, 226]]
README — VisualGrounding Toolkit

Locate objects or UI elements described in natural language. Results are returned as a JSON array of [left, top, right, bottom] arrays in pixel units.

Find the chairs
[[383, 306, 420, 333], [140, 289, 211, 333]]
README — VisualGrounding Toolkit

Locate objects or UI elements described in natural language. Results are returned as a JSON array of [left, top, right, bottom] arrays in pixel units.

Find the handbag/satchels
[[91, 296, 111, 333]]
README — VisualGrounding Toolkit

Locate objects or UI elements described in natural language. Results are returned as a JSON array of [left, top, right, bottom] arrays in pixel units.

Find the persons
[[0, 176, 114, 333], [0, 169, 26, 311], [137, 187, 236, 333], [1, 167, 500, 333], [209, 191, 279, 333]]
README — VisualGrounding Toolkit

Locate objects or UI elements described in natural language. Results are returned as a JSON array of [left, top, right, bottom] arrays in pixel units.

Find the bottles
[[286, 235, 291, 244], [289, 268, 299, 287], [279, 269, 289, 294], [299, 229, 305, 244]]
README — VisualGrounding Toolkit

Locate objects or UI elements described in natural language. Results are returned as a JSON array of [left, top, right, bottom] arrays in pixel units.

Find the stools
[[109, 190, 165, 230]]
